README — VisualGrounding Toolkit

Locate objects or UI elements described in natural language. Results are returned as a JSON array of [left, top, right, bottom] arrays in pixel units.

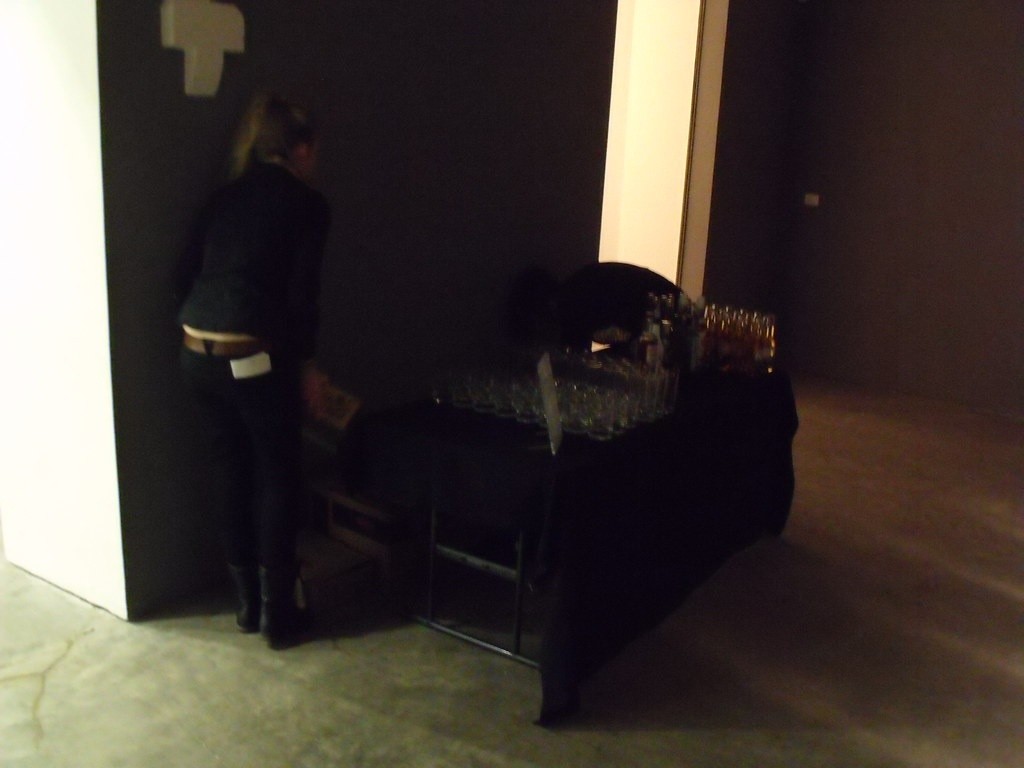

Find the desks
[[316, 331, 800, 729]]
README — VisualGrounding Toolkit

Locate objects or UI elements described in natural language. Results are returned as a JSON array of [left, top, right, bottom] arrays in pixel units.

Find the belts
[[180, 332, 264, 356]]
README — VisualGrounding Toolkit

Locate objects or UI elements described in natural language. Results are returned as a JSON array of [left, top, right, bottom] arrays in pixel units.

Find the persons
[[174, 99, 340, 643]]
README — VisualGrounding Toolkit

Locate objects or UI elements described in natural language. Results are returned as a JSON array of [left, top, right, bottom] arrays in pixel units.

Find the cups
[[450, 366, 636, 442]]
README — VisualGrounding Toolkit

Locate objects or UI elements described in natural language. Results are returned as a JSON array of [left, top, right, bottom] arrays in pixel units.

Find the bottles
[[625, 319, 676, 418], [642, 292, 777, 381]]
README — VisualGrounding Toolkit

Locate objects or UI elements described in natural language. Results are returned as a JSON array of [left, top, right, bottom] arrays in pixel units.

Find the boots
[[227, 562, 322, 644]]
